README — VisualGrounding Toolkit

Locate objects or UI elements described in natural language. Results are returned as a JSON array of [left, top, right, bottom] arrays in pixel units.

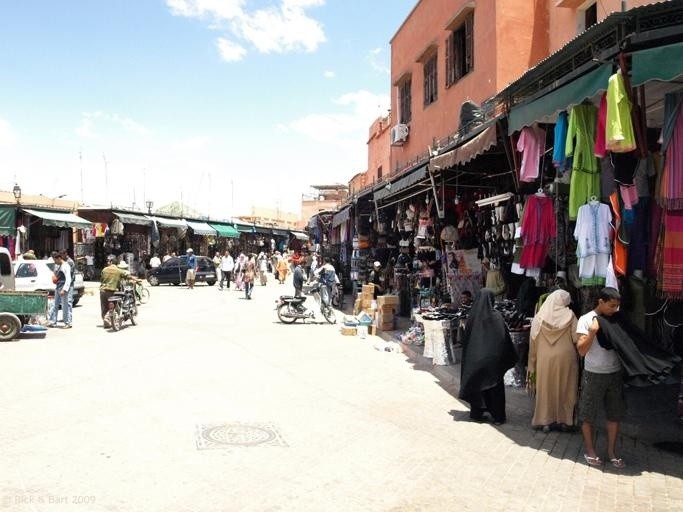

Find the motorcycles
[[134, 279, 150, 304], [331, 282, 345, 307], [107, 277, 140, 330], [273, 284, 338, 326]]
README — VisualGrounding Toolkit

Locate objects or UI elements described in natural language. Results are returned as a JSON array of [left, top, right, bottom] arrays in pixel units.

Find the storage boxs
[[341, 284, 399, 336]]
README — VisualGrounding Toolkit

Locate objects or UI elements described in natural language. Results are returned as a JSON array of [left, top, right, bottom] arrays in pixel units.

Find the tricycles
[[0, 290, 49, 341]]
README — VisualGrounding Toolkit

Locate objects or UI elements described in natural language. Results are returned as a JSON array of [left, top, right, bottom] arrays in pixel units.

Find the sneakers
[[63, 324, 73, 328], [103, 316, 113, 328]]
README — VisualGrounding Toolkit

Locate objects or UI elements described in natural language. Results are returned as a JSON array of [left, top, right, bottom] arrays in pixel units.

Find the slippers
[[583, 453, 601, 467], [609, 455, 627, 470]]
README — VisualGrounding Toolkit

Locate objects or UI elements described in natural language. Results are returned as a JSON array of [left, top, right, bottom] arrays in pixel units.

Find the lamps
[[455, 166, 460, 205]]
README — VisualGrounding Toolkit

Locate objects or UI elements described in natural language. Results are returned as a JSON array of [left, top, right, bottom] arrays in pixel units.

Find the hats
[[22, 250, 36, 259], [186, 247, 194, 255], [373, 261, 382, 267]]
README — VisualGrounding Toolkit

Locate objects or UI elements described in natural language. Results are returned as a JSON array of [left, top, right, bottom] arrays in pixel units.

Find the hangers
[[476, 192, 514, 208], [589, 195, 599, 206], [535, 188, 545, 198]]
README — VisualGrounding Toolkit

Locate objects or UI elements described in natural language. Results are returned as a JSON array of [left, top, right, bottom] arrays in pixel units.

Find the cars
[[147, 255, 218, 288], [13, 260, 85, 308]]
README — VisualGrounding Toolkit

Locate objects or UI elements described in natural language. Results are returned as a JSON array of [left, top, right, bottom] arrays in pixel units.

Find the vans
[[1, 248, 15, 292]]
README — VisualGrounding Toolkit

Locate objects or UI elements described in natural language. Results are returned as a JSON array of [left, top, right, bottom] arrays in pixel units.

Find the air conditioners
[[390, 124, 409, 147]]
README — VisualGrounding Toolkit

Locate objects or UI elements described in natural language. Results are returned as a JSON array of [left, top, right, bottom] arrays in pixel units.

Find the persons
[[577, 287, 625, 470], [44, 249, 75, 327], [234, 253, 245, 291], [277, 251, 282, 260], [292, 260, 309, 301], [162, 252, 172, 265], [100, 254, 137, 326], [269, 252, 279, 280], [244, 255, 256, 299], [218, 250, 234, 290], [369, 261, 384, 298], [455, 252, 466, 269], [212, 251, 221, 286], [456, 290, 474, 344], [317, 256, 335, 306], [276, 256, 287, 284], [460, 289, 517, 424], [171, 251, 176, 256], [185, 247, 197, 289], [116, 260, 129, 287], [84, 250, 97, 279], [53, 253, 73, 329], [528, 290, 578, 434], [48, 250, 59, 264], [256, 250, 268, 286], [273, 250, 277, 256], [149, 252, 161, 270], [446, 252, 456, 270]]
